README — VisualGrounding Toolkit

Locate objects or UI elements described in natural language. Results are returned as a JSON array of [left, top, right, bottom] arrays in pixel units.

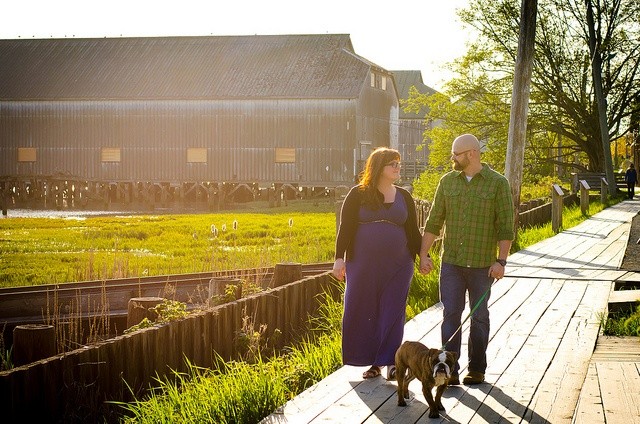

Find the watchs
[[497, 259, 506, 266]]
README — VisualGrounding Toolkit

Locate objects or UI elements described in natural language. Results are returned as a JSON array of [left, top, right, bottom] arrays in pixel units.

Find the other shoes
[[448, 374, 460, 385], [463, 370, 484, 385]]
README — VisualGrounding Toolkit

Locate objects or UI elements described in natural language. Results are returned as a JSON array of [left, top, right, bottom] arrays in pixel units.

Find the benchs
[[577, 172, 629, 188]]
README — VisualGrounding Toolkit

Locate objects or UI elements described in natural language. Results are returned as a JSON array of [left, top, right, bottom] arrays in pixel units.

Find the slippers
[[386, 367, 397, 380], [362, 366, 381, 378]]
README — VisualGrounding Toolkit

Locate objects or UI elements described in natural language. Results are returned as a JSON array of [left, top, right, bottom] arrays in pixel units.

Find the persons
[[333, 148, 433, 379], [419, 134, 515, 386], [625, 164, 638, 199]]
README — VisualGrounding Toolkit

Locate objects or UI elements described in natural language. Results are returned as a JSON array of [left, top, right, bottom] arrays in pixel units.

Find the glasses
[[451, 149, 472, 157], [385, 161, 402, 168]]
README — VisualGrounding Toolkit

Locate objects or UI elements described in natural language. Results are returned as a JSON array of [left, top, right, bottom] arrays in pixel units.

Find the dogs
[[392, 339, 460, 420]]
